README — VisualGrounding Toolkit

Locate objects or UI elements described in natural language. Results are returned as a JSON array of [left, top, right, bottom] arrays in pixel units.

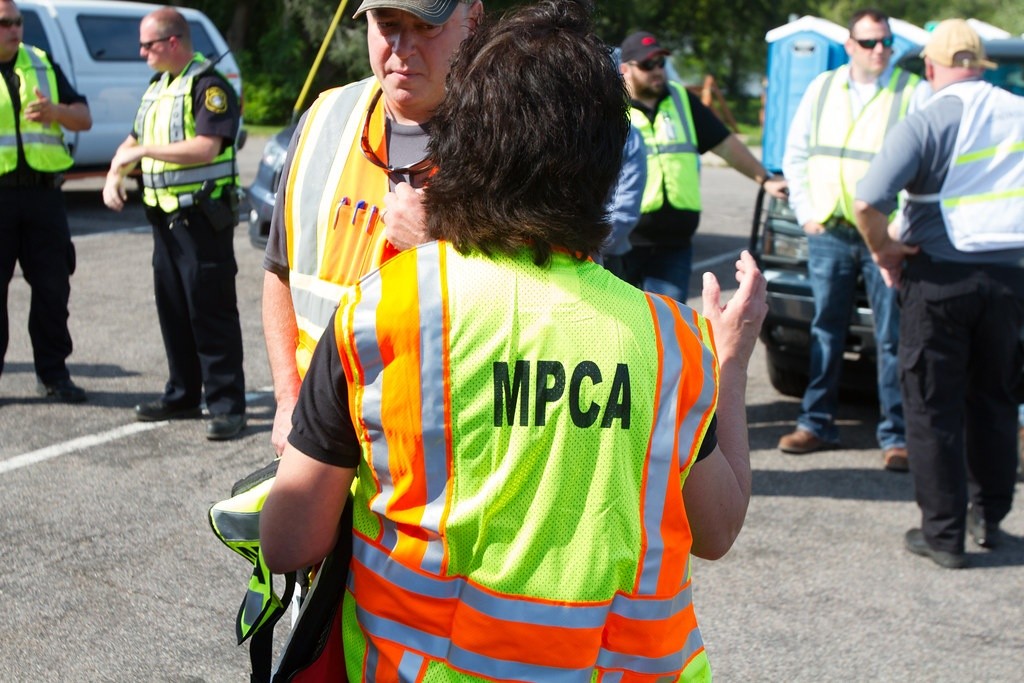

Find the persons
[[103, 8, 247, 441], [0, 0, 93, 403], [778, 9, 934, 472], [854, 18, 1024, 569], [258, 0, 789, 683]]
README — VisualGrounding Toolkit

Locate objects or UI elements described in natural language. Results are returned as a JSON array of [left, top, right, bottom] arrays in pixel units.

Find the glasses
[[0, 16, 24, 28], [852, 34, 895, 48], [630, 56, 666, 72], [139, 35, 182, 51]]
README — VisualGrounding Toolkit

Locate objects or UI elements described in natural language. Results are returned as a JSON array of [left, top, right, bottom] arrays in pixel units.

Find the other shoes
[[206, 411, 246, 438], [905, 528, 967, 568], [47, 376, 86, 403], [778, 429, 826, 452], [134, 398, 201, 421], [884, 446, 908, 473], [965, 501, 1000, 547]]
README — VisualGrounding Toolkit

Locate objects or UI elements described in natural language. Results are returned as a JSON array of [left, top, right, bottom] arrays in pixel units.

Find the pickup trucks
[[15, 0, 247, 196], [746, 166, 876, 400]]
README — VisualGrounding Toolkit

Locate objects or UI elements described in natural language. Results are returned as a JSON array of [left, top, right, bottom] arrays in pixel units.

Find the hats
[[352, 0, 460, 27], [620, 32, 672, 63], [919, 18, 999, 73]]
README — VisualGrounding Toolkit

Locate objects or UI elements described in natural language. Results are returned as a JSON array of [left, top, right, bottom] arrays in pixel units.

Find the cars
[[247, 124, 302, 250]]
